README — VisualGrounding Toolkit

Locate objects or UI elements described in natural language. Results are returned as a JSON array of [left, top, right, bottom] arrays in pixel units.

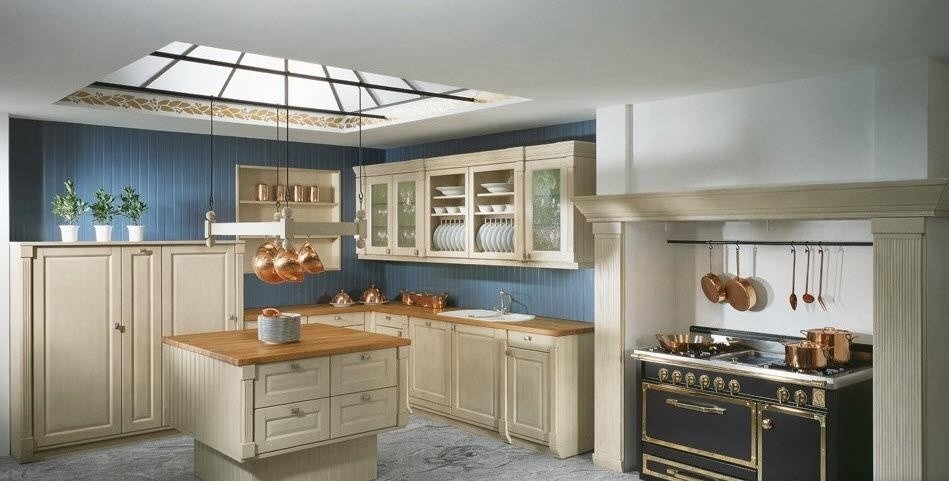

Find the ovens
[[639, 378, 874, 481]]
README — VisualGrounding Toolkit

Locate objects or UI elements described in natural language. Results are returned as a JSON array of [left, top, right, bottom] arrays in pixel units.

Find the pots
[[784, 341, 833, 371], [725, 248, 757, 313], [252, 238, 325, 287], [800, 327, 858, 365], [701, 248, 726, 303], [657, 334, 715, 354]]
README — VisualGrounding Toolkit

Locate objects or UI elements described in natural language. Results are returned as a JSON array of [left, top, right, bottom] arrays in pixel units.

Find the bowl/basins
[[480, 183, 514, 193], [433, 205, 514, 214], [436, 186, 465, 196]]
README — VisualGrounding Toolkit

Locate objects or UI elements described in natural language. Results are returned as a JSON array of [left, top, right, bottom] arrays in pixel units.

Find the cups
[[400, 288, 449, 309], [258, 183, 319, 203]]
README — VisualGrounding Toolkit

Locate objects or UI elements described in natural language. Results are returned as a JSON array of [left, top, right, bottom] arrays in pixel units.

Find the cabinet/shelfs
[[453, 318, 506, 442], [235, 163, 342, 272], [11, 240, 245, 464], [352, 158, 425, 262], [524, 140, 596, 270], [504, 325, 594, 459], [425, 146, 524, 267], [368, 306, 409, 335], [307, 307, 368, 330], [409, 312, 452, 419], [243, 312, 306, 330]]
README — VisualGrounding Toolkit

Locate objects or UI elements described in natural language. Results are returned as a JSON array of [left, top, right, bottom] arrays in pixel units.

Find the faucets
[[494, 287, 506, 314]]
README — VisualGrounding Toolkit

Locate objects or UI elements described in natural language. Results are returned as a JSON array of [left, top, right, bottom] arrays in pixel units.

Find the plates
[[256, 312, 301, 347], [330, 303, 356, 308], [357, 300, 389, 306], [432, 223, 517, 254]]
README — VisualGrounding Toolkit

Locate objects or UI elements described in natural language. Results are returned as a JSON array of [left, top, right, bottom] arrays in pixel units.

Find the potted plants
[[50, 177, 89, 241], [120, 184, 148, 241], [89, 185, 116, 241]]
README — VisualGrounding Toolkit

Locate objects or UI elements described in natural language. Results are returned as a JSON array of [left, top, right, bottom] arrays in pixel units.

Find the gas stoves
[[630, 333, 872, 412]]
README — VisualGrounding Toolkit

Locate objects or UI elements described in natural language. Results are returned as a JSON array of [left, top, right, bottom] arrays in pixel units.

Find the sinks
[[485, 313, 536, 324], [436, 308, 500, 320]]
